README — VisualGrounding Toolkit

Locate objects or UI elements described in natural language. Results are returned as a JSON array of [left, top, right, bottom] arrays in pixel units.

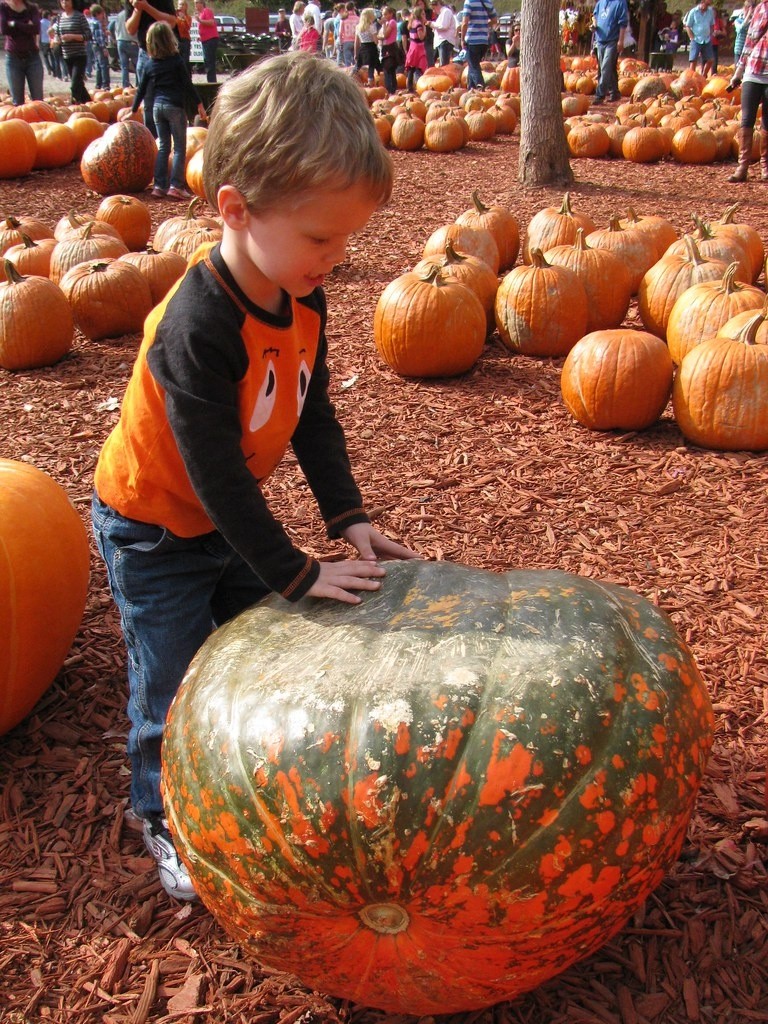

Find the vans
[[268, 15, 291, 32], [214, 15, 246, 32]]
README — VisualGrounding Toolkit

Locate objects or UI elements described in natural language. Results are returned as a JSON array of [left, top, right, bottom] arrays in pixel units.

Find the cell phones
[[726, 79, 742, 93]]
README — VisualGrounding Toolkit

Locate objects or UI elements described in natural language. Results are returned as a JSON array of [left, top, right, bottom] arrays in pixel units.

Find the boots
[[728, 128, 753, 183], [760, 126, 768, 178]]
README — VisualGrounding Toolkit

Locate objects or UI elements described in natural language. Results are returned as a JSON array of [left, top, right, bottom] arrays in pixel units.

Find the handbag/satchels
[[50, 16, 61, 48]]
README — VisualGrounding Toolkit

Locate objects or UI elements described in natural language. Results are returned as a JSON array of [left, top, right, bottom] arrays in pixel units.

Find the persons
[[275, 0, 503, 104], [728, 0, 768, 184], [92, 50, 424, 901], [193, 0, 220, 83], [588, 0, 629, 105], [646, 0, 757, 78], [505, 23, 520, 69], [0, 0, 45, 106], [120, 19, 207, 201], [460, 0, 498, 93], [40, 0, 192, 96]]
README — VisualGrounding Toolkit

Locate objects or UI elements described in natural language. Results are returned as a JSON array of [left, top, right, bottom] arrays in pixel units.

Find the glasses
[[514, 29, 520, 32]]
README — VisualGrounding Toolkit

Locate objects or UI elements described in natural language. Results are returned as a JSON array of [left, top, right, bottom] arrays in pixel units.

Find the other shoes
[[607, 92, 622, 102], [592, 98, 603, 105], [95, 85, 110, 90]]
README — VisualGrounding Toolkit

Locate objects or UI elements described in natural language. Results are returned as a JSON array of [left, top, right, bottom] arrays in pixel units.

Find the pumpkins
[[161, 554, 714, 1017], [340, 54, 762, 164], [374, 188, 768, 452], [0, 83, 208, 199], [0, 194, 223, 368], [0, 456, 91, 737]]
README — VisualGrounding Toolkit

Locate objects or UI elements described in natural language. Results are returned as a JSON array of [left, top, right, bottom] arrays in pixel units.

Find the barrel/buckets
[[648, 50, 674, 71]]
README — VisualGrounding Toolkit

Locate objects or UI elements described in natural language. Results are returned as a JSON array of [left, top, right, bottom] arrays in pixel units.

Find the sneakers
[[167, 184, 190, 199], [151, 184, 169, 198], [141, 818, 199, 900]]
